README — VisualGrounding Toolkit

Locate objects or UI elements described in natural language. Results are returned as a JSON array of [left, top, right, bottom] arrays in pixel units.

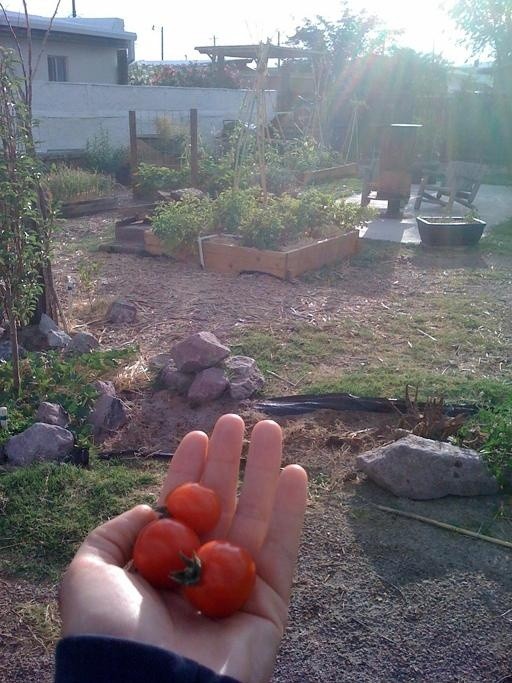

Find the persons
[[52, 413, 309, 681]]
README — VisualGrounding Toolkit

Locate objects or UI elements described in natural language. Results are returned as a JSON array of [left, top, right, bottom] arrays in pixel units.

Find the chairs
[[413, 162, 488, 210]]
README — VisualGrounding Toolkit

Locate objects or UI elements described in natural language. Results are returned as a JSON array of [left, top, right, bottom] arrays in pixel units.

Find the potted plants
[[415, 208, 487, 246]]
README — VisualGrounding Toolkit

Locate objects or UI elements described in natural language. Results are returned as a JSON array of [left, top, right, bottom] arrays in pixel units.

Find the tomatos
[[168, 539, 257, 619], [155, 482, 221, 535], [132, 517, 200, 592]]
